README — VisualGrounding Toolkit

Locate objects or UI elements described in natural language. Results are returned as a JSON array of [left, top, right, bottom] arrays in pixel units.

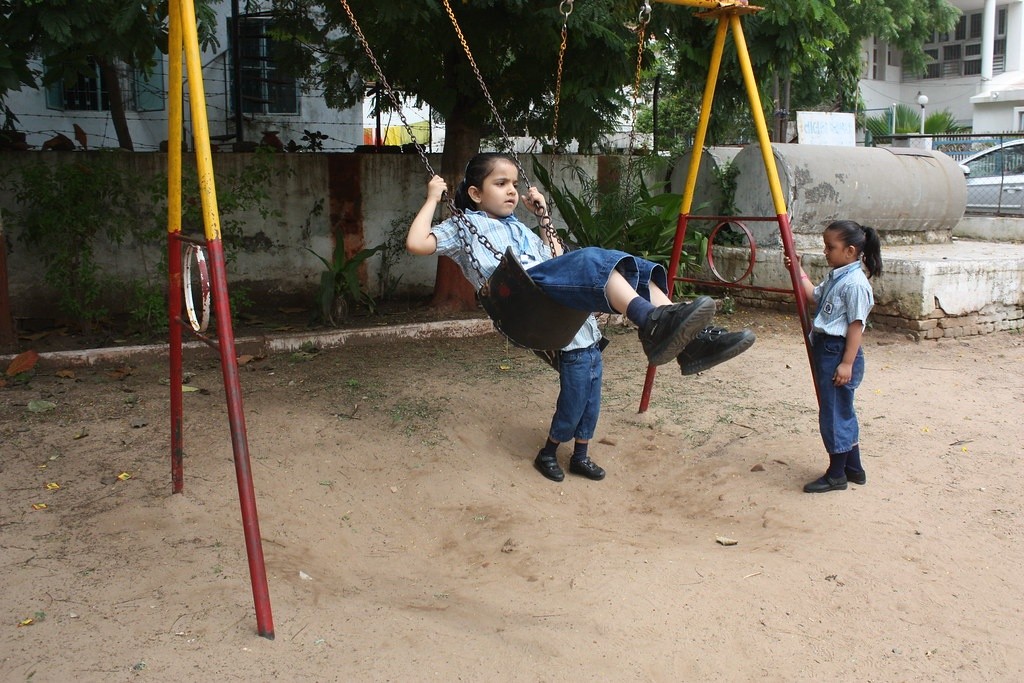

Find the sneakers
[[535, 448, 564, 481], [638, 295, 716, 365], [677, 326, 756, 375], [570, 455, 605, 480]]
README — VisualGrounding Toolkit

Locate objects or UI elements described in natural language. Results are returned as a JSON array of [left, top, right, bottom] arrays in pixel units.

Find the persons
[[405, 152, 756, 375], [533, 307, 606, 483], [784, 220, 885, 493]]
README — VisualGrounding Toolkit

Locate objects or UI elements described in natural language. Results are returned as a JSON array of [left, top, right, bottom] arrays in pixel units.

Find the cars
[[955, 137, 1024, 216]]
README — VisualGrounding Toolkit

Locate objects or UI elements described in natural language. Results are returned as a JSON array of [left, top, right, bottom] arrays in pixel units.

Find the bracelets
[[800, 274, 808, 278]]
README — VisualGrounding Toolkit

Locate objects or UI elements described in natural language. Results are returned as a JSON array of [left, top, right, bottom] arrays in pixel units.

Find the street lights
[[918, 95, 928, 134]]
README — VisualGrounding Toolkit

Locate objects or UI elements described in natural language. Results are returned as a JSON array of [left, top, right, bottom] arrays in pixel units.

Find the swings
[[536, 0, 653, 375], [340, 0, 593, 352]]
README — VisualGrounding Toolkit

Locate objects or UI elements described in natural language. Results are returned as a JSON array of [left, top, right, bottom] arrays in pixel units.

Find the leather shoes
[[827, 466, 866, 484], [804, 473, 847, 492]]
